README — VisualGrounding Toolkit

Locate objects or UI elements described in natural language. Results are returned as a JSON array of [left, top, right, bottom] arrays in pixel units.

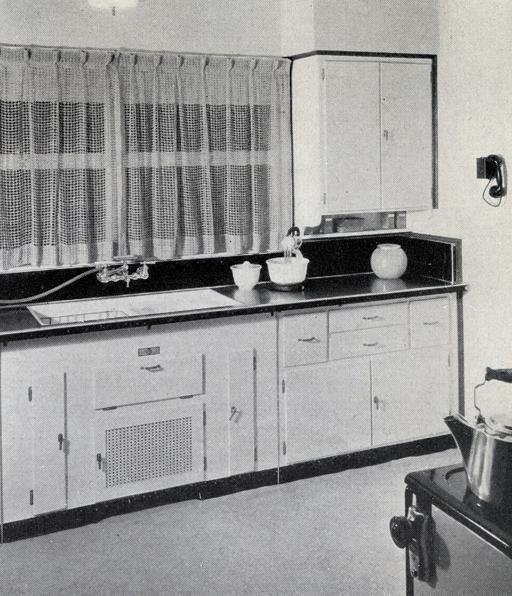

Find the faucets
[[96, 255, 158, 288]]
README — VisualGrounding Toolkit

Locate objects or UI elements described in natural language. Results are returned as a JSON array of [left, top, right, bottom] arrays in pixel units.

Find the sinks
[[25, 286, 246, 326]]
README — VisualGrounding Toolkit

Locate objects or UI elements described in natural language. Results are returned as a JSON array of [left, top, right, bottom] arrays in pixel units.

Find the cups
[[230, 264, 261, 291]]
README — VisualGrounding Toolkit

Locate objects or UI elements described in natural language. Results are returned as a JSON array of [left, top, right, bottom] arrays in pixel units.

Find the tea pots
[[444, 366, 511, 510]]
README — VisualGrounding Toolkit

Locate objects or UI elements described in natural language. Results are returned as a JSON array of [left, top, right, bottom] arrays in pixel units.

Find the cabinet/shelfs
[[291, 55, 434, 216]]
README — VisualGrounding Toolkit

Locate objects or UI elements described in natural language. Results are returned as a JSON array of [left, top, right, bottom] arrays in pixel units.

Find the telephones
[[487, 155, 505, 200]]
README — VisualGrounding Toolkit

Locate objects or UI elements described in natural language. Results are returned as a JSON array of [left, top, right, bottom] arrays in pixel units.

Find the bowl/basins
[[266, 256, 310, 285]]
